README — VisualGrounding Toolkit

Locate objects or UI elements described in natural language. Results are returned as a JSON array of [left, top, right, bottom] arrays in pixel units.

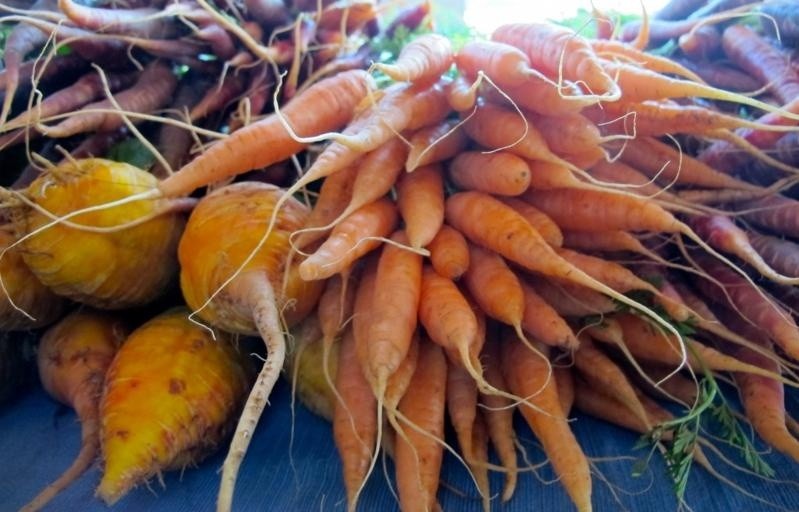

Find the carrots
[[0, 1, 798, 512]]
